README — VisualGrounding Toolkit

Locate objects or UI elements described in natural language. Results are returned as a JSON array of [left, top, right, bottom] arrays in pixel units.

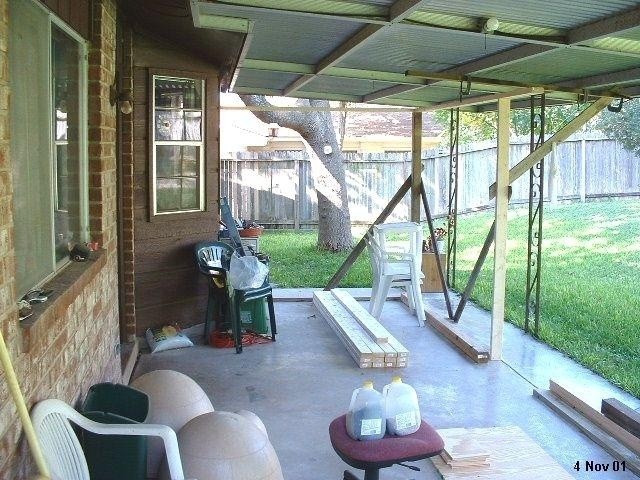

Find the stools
[[330, 414, 445, 480]]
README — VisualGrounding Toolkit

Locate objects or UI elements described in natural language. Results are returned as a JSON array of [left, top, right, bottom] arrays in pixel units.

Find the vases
[[434, 240, 444, 253]]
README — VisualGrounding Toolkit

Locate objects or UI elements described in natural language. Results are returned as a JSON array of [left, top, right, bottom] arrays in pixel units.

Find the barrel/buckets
[[382, 376, 421, 436], [345, 381, 386, 441]]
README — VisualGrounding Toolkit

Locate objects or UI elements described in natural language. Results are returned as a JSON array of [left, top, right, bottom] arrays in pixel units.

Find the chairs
[[367, 223, 428, 328], [29, 399, 185, 480], [193, 240, 277, 353]]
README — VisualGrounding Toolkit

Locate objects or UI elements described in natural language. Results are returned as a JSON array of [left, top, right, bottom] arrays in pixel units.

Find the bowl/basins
[[238, 228, 260, 238]]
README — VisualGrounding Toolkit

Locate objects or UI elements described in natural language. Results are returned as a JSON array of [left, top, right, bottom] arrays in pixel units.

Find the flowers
[[431, 227, 447, 241]]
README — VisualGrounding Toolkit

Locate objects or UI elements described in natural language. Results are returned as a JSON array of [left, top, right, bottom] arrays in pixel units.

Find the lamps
[[110, 86, 134, 114]]
